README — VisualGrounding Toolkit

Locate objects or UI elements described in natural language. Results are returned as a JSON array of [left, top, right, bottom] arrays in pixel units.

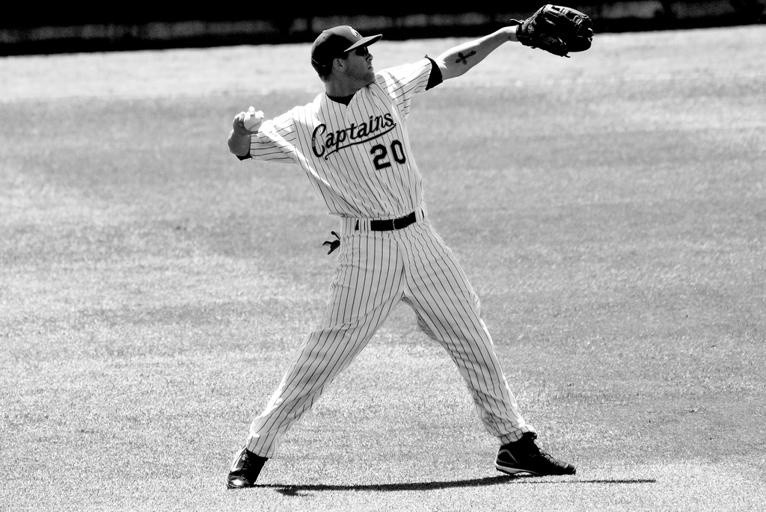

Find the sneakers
[[226, 444, 266, 489], [493, 430, 576, 478]]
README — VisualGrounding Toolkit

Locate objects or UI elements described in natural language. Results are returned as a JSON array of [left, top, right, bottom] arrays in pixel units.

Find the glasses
[[354, 46, 369, 57]]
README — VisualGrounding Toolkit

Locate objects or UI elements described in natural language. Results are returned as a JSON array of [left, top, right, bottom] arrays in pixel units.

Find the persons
[[224, 4, 595, 490]]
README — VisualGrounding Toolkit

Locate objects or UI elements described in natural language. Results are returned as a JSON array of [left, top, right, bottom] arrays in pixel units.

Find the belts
[[354, 208, 424, 231]]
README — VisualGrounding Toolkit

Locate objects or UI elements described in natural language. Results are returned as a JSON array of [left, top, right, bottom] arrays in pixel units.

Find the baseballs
[[243, 112, 262, 131]]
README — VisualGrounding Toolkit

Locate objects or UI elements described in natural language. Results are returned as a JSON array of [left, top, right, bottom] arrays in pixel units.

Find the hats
[[310, 25, 383, 73]]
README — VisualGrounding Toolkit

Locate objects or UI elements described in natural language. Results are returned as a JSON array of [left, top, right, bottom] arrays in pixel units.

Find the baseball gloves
[[510, 4, 594, 57]]
